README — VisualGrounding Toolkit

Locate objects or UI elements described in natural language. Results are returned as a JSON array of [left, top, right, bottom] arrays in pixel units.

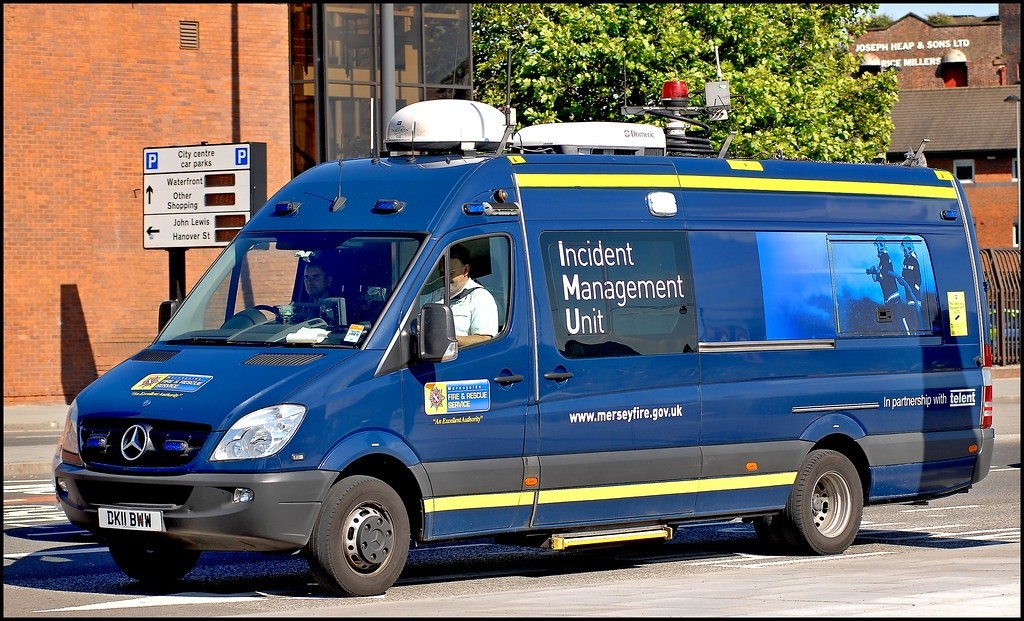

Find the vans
[[54, 80, 995, 599]]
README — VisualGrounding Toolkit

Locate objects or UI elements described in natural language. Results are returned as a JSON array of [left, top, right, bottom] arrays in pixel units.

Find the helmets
[[873, 237, 889, 252], [901, 237, 914, 255]]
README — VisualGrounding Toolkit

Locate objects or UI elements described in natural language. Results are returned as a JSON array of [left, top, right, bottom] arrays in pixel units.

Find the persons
[[417, 244, 498, 347], [289, 259, 355, 325]]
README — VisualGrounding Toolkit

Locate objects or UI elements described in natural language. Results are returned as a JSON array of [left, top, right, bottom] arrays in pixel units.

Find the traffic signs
[[139, 142, 266, 247]]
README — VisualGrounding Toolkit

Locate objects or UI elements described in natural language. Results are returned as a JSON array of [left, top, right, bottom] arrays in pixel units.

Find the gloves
[[870, 266, 882, 274], [872, 274, 879, 282]]
